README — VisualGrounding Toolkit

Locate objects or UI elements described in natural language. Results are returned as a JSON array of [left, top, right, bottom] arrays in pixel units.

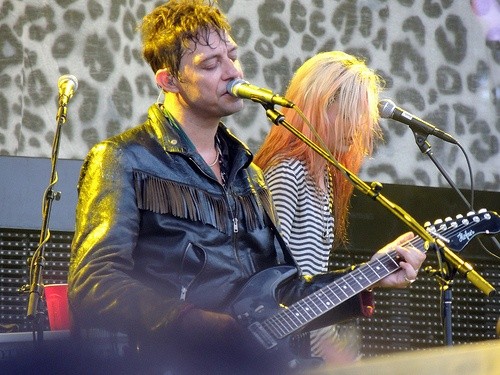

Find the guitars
[[228, 207, 500, 375]]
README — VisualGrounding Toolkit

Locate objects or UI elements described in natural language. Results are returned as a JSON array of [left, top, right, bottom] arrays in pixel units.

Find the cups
[[43, 284, 69, 331]]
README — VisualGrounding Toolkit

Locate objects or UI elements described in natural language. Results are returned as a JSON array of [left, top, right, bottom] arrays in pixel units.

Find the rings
[[404, 276, 415, 285]]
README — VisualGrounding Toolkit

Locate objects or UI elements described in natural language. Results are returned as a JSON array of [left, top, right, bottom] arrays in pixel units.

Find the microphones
[[377, 99, 458, 144], [227, 79, 295, 107], [57, 74, 79, 124]]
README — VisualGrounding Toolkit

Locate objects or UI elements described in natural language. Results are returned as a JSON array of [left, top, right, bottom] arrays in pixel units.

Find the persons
[[252, 50, 384, 367], [67, 1, 427, 370]]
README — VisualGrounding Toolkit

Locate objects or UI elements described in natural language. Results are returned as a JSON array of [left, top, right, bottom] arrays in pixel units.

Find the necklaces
[[209, 149, 220, 167]]
